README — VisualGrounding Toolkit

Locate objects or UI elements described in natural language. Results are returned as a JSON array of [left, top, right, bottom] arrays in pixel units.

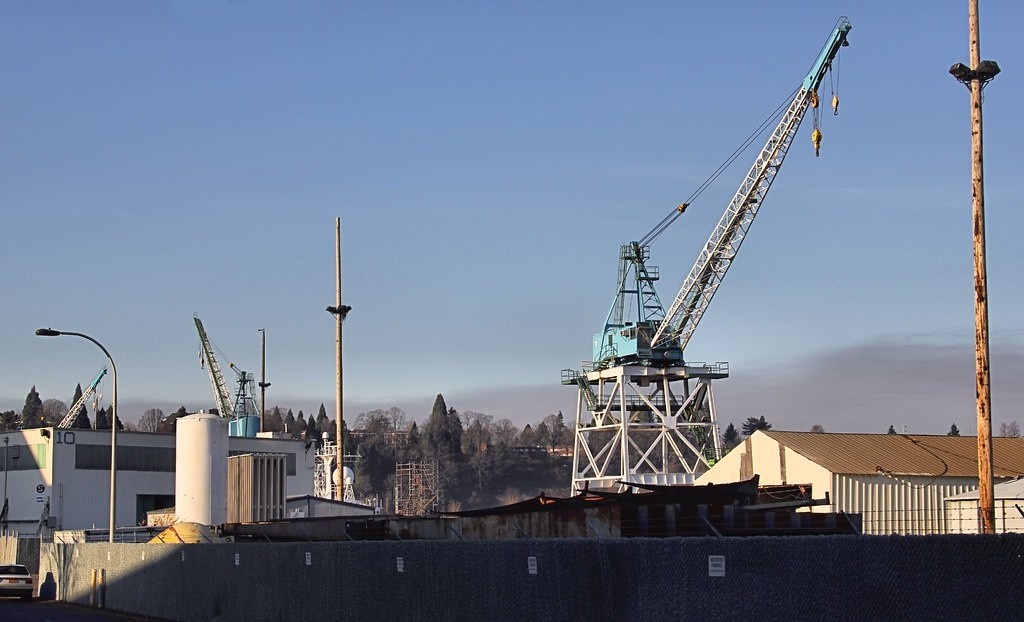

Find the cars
[[0, 564, 34, 601]]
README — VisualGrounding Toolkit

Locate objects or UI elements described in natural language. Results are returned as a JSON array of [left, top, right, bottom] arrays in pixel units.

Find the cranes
[[57, 366, 109, 429], [560, 15, 852, 499], [193, 311, 261, 438]]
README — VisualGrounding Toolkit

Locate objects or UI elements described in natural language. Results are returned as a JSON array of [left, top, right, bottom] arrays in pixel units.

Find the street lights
[[36, 328, 117, 544], [258, 328, 266, 432]]
